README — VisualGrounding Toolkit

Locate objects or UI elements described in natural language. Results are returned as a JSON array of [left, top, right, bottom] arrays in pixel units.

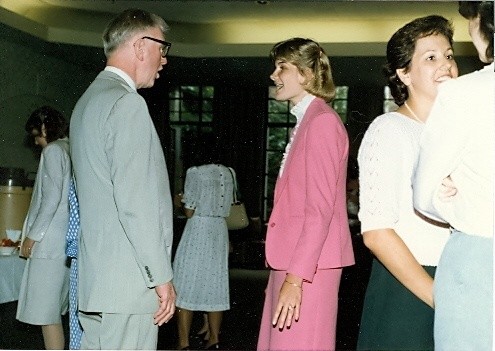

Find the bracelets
[[284, 279, 302, 288]]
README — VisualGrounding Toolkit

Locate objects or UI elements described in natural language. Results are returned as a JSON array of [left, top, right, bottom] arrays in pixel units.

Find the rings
[[28, 248, 31, 250]]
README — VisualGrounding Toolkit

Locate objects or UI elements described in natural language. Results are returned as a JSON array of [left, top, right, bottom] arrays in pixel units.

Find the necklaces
[[404, 101, 423, 122]]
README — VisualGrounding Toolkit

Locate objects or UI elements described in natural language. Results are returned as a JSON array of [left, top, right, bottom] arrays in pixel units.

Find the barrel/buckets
[[0, 178, 34, 246]]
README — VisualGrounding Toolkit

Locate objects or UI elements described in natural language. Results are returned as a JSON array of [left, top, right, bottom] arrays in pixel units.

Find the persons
[[20, 104, 77, 350], [68, 8, 178, 350], [359, 13, 462, 351], [254, 37, 356, 351], [411, 0, 494, 351], [170, 137, 238, 351]]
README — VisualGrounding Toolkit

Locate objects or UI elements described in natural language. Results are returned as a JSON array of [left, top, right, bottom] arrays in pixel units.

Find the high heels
[[192, 330, 208, 346]]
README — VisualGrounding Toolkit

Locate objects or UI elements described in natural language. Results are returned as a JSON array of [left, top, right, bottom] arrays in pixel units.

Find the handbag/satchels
[[225, 167, 249, 230]]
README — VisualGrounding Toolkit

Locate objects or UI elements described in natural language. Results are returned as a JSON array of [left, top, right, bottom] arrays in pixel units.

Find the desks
[[0, 242, 28, 305]]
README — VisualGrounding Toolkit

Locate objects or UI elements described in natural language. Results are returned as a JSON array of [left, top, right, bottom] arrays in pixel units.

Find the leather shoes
[[181, 342, 220, 351]]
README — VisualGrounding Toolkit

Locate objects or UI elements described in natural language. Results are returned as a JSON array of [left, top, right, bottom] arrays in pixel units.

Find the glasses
[[141, 36, 172, 58]]
